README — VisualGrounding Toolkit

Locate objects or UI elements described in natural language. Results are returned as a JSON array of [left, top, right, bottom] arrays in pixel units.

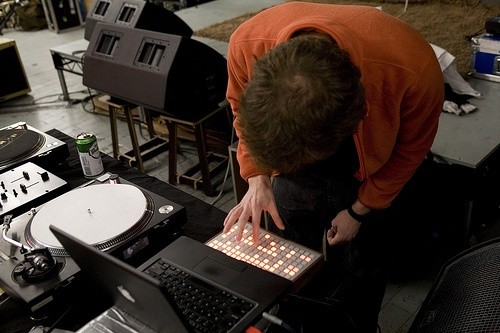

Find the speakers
[[82, 22, 229, 113], [407, 236, 500, 333], [84, 0, 194, 42]]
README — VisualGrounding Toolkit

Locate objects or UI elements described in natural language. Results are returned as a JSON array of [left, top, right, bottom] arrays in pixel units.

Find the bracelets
[[348, 206, 368, 222]]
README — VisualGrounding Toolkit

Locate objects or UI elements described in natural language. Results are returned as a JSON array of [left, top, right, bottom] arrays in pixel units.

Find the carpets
[[192, 0, 500, 80], [0, 126, 325, 333]]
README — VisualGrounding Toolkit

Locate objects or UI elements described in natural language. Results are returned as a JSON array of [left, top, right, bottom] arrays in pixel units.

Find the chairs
[[409, 235, 500, 333]]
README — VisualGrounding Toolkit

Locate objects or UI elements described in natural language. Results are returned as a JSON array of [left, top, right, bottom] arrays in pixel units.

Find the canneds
[[76, 132, 105, 180]]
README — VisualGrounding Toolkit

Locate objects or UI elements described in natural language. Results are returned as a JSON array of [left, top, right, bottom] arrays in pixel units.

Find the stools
[[98, 94, 172, 175], [161, 103, 235, 199]]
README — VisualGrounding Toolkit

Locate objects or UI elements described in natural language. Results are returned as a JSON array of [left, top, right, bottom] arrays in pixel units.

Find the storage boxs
[[41, 0, 84, 33], [0, 37, 32, 104]]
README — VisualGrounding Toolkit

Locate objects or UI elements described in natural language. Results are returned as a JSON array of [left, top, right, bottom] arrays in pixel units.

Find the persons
[[224, 0, 445, 333]]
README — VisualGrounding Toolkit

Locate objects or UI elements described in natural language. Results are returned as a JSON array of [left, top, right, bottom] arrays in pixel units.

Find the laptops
[[49, 224, 289, 333]]
